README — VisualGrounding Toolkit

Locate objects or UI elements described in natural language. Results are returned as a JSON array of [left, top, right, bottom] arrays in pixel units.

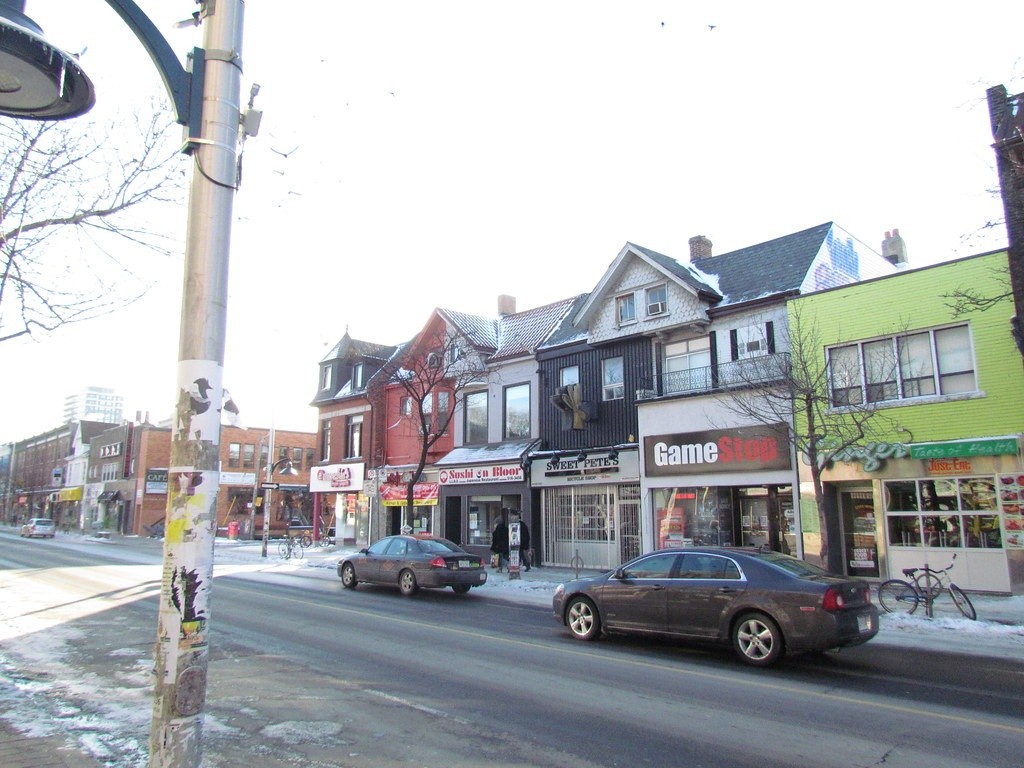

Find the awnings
[[98, 490, 118, 503], [61, 487, 81, 501], [228, 487, 265, 498], [280, 484, 309, 492]]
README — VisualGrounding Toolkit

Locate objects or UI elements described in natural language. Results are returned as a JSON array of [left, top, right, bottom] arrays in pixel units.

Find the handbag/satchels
[[490, 553, 499, 568]]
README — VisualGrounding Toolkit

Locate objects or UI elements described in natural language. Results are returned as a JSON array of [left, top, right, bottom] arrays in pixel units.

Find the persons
[[520, 516, 531, 572], [63, 519, 70, 535], [490, 518, 510, 573]]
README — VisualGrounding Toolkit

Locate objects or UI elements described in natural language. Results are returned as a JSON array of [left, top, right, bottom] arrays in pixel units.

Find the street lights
[[0, 0, 261, 768], [3, 440, 16, 525], [261, 458, 298, 557]]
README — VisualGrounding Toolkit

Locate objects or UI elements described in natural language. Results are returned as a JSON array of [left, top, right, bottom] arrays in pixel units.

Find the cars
[[337, 535, 487, 596], [553, 547, 879, 667], [20, 519, 55, 538], [322, 527, 336, 544]]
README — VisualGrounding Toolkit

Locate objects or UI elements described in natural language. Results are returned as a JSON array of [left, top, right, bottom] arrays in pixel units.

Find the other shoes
[[526, 567, 531, 571]]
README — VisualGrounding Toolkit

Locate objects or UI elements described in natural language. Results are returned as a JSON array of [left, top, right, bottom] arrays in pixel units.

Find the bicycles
[[278, 535, 304, 559], [878, 553, 977, 621], [300, 531, 331, 548]]
